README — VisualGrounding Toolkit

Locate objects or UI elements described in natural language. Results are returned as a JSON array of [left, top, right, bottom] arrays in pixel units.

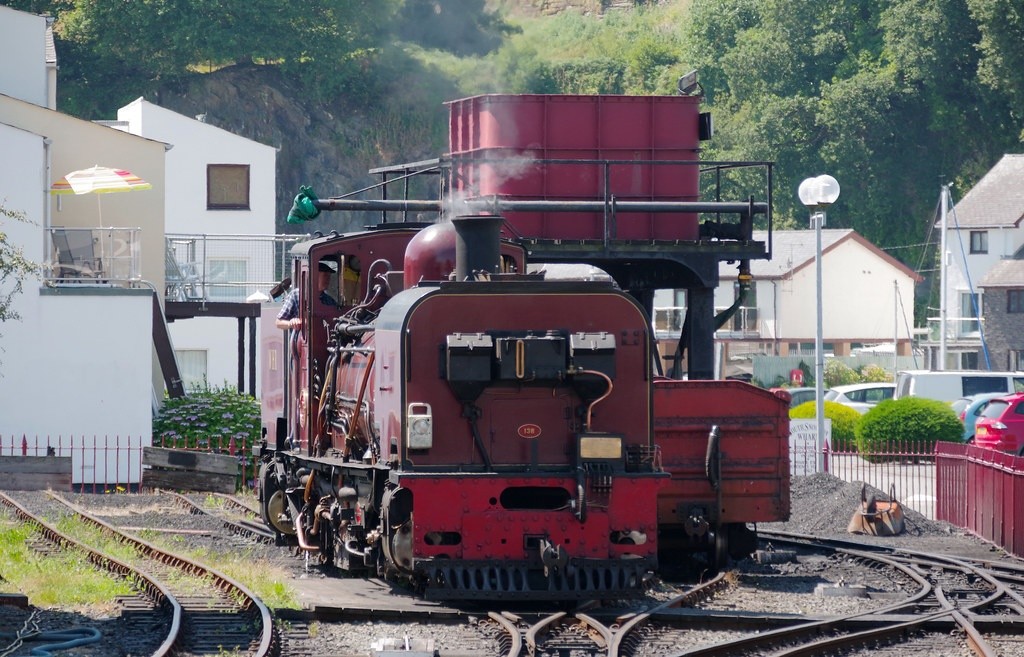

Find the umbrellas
[[49, 163, 153, 271]]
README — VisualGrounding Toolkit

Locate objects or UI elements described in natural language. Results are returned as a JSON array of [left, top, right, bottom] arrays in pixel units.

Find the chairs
[[50, 226, 102, 283], [165, 236, 210, 302]]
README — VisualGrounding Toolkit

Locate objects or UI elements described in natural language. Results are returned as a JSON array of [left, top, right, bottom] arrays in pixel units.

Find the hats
[[318, 261, 338, 273]]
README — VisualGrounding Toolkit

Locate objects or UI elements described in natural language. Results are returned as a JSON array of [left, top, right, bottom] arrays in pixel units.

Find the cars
[[824, 383, 897, 417], [784, 387, 829, 411], [949, 390, 1009, 445], [973, 392, 1024, 457]]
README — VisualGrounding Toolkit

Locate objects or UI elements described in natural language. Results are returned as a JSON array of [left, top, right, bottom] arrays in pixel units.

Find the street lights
[[797, 174, 842, 473]]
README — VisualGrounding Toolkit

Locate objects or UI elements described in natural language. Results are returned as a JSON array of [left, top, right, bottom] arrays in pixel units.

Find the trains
[[249, 92, 791, 602]]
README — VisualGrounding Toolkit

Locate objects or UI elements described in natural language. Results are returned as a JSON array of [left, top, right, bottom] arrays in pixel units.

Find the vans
[[893, 369, 1024, 409]]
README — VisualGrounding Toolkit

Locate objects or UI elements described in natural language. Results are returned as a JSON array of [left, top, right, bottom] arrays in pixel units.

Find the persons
[[276, 262, 339, 330]]
